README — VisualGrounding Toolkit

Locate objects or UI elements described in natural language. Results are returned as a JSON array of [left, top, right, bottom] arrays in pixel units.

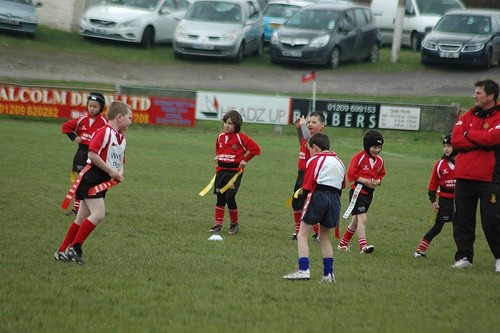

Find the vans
[[369, 0, 475, 53]]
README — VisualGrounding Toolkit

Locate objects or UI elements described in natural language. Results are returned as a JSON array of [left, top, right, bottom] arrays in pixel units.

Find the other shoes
[[64, 209, 77, 216], [312, 234, 319, 241], [292, 233, 297, 241]]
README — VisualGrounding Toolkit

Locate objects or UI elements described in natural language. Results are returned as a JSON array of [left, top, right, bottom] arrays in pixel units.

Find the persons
[[449, 79, 500, 272], [61, 92, 108, 217], [199, 110, 261, 235], [54, 101, 133, 265], [414, 134, 457, 258], [288, 111, 340, 240], [283, 133, 346, 282], [337, 130, 386, 254]]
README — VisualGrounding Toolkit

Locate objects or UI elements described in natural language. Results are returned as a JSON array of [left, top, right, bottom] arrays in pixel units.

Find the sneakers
[[54, 248, 69, 261], [282, 269, 310, 280], [228, 223, 240, 235], [495, 259, 500, 272], [450, 257, 472, 268], [337, 244, 352, 252], [322, 273, 335, 283], [65, 243, 85, 265], [360, 245, 374, 254], [210, 226, 221, 232], [415, 251, 427, 258]]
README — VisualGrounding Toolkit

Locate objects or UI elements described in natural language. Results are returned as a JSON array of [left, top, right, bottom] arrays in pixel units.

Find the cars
[[78, 0, 193, 49], [421, 8, 500, 71], [262, 0, 315, 45], [172, 0, 265, 64], [270, 3, 383, 70], [0, 0, 43, 40]]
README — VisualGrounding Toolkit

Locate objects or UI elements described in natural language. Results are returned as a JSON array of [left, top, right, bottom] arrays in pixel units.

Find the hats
[[443, 134, 456, 158], [86, 93, 105, 115], [363, 131, 384, 156]]
